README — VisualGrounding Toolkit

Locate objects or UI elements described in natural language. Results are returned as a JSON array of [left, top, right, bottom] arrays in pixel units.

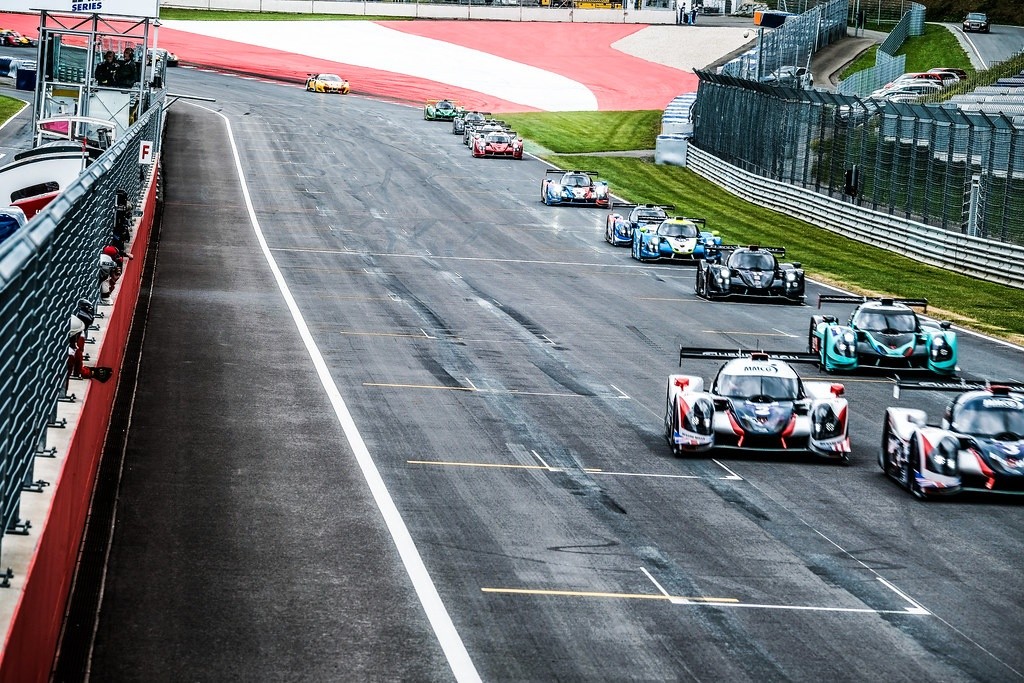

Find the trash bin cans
[[16, 61, 36, 90]]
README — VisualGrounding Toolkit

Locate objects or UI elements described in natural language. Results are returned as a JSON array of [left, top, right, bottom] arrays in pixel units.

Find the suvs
[[962, 12, 990, 33]]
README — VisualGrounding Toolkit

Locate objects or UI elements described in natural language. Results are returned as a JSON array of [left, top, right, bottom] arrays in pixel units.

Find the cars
[[769, 66, 814, 89], [840, 68, 968, 119]]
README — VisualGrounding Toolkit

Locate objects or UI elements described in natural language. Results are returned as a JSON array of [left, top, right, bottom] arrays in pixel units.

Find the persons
[[678, 3, 699, 25], [96, 48, 138, 90], [70, 223, 135, 382]]
